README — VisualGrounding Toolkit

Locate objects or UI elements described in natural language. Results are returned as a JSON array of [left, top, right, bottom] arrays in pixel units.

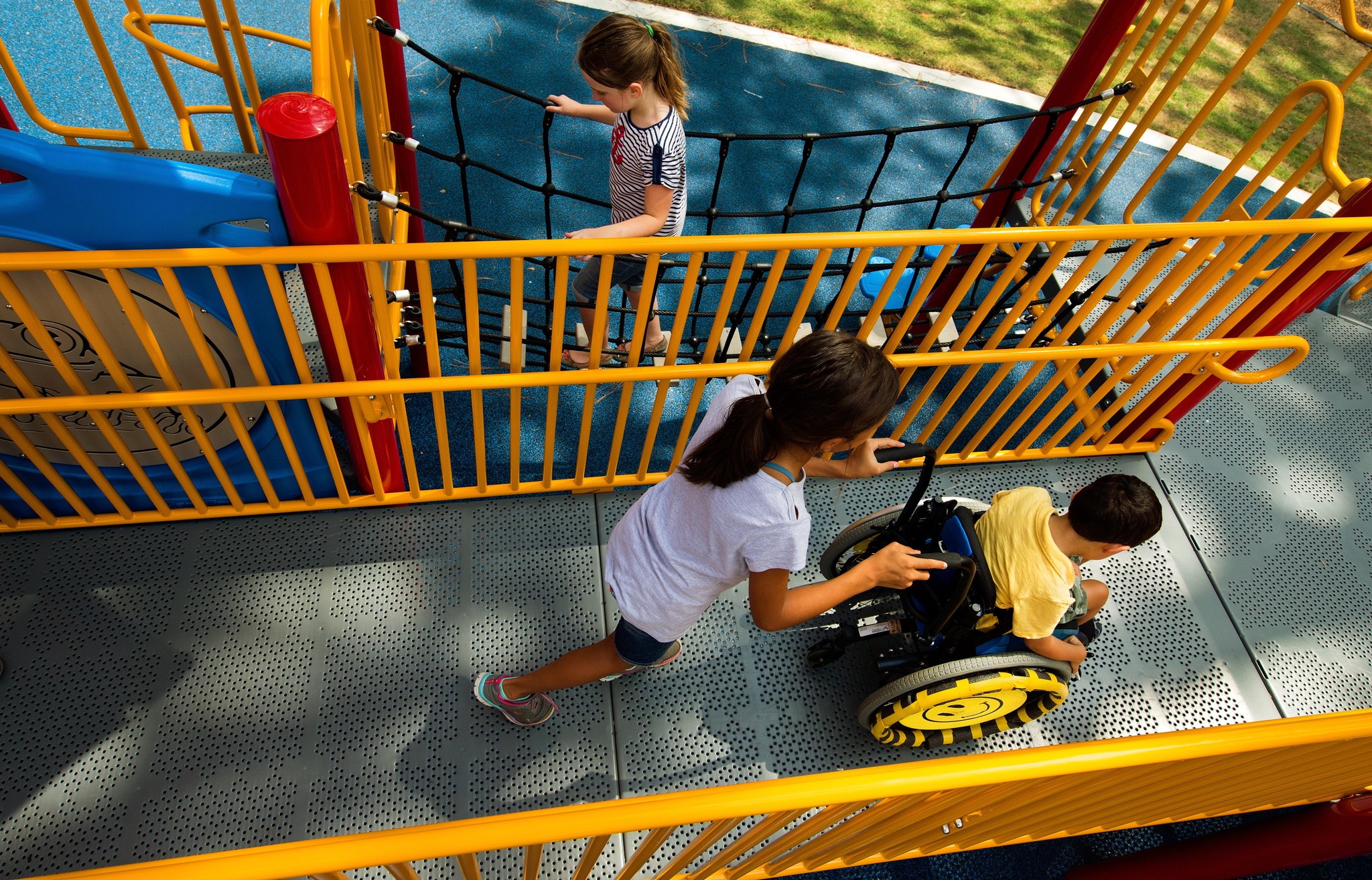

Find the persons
[[544, 13, 691, 368], [973, 474, 1163, 675], [474, 330, 949, 725]]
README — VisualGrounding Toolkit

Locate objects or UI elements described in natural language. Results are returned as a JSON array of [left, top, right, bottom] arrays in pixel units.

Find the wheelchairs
[[806, 443, 1104, 750]]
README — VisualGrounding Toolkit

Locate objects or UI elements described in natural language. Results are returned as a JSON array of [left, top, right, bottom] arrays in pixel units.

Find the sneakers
[[599, 639, 682, 681], [475, 672, 558, 726]]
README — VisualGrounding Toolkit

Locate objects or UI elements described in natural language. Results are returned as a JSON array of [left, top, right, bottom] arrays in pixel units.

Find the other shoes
[[561, 345, 612, 368], [613, 334, 667, 362]]
